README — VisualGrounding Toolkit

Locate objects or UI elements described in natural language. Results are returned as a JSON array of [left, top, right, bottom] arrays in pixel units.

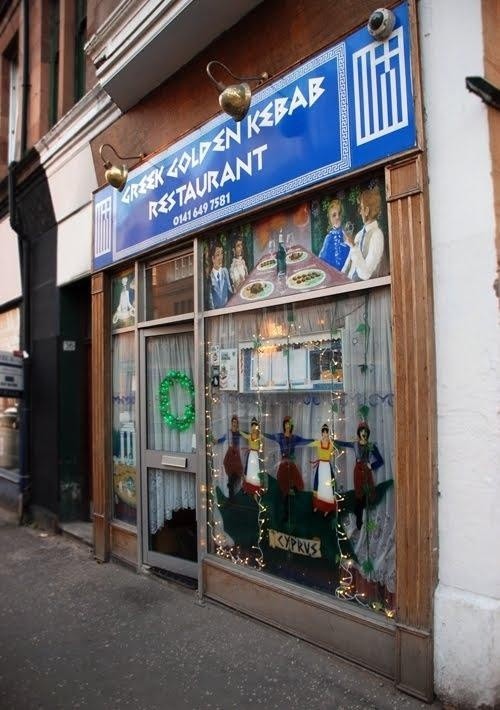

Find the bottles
[[277, 231, 288, 278]]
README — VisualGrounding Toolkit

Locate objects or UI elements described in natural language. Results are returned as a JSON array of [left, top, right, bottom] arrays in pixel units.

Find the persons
[[341, 185, 389, 281], [319, 200, 353, 270], [205, 238, 235, 309], [213, 415, 248, 500], [229, 234, 250, 289], [260, 417, 317, 521], [237, 417, 264, 500], [331, 420, 384, 530], [306, 424, 343, 519]]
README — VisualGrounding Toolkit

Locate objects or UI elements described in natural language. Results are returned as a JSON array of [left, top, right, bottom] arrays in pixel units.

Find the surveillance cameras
[[367, 8, 396, 41]]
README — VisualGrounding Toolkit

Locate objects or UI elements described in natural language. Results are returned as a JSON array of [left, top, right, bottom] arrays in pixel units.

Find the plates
[[256, 258, 278, 271], [286, 251, 308, 265], [240, 281, 275, 300], [286, 268, 326, 290]]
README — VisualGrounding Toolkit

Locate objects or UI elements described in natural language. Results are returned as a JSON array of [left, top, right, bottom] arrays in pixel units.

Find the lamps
[[96, 142, 148, 196], [203, 59, 271, 127]]
